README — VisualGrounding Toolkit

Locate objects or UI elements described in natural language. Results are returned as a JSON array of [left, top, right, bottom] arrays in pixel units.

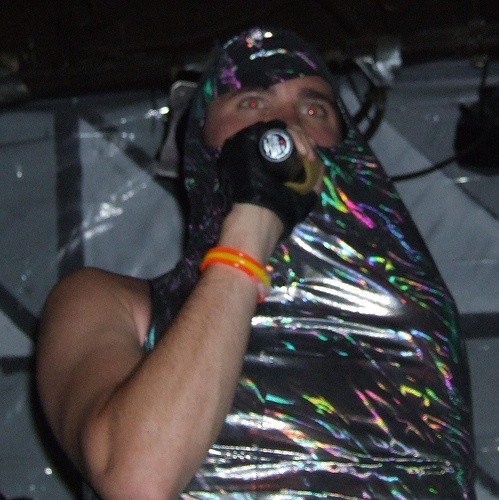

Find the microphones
[[255, 126, 297, 176]]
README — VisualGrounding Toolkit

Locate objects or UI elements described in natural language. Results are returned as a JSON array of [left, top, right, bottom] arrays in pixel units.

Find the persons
[[34, 20, 480, 500]]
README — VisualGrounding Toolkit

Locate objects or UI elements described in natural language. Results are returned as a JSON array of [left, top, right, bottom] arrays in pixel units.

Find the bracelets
[[199, 245, 271, 304]]
